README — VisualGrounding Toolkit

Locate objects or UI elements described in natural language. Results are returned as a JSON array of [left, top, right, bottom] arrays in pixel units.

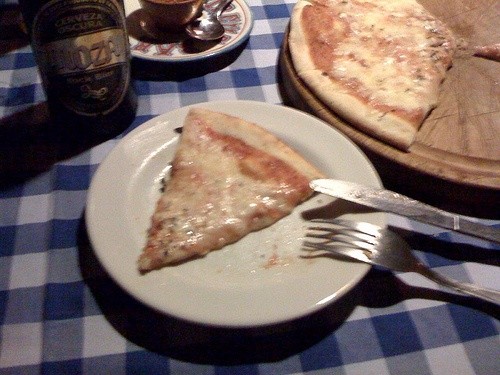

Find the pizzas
[[137, 108, 324, 272], [288, 0, 456, 151]]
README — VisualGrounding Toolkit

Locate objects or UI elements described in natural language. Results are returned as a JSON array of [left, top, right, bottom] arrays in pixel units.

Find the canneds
[[19, 0, 138, 143]]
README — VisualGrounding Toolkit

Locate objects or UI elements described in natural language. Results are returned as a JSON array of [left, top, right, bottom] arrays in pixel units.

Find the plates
[[84, 100, 387, 330], [124, 0, 253, 63]]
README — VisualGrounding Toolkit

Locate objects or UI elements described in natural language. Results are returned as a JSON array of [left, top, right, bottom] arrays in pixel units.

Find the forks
[[300, 220, 500, 314]]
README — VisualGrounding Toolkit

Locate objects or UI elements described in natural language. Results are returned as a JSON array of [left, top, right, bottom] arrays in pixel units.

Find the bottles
[[20, 1, 140, 138]]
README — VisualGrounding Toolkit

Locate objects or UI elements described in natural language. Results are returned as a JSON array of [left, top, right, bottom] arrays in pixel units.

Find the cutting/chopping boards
[[279, 0, 500, 218]]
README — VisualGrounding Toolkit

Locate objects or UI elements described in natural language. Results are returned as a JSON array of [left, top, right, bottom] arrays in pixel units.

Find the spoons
[[188, 1, 235, 41]]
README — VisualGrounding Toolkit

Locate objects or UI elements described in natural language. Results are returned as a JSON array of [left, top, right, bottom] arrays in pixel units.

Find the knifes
[[309, 176, 499, 243]]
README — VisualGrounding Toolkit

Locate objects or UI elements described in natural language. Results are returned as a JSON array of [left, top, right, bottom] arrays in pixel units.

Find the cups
[[132, 0, 202, 39]]
